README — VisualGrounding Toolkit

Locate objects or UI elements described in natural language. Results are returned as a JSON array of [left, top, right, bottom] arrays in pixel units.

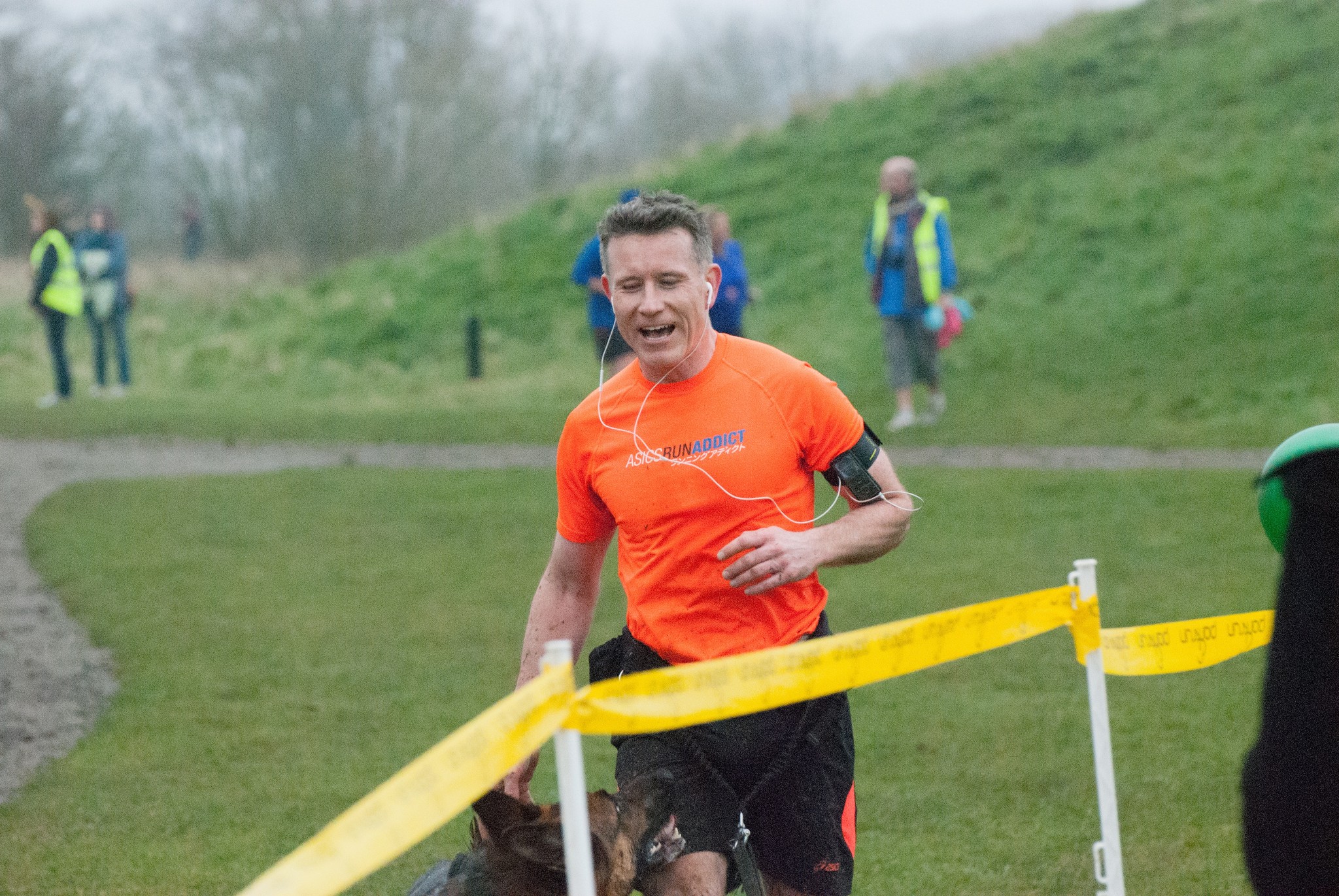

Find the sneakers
[[88, 386, 109, 398], [109, 384, 126, 399], [924, 397, 951, 430], [35, 394, 64, 409], [887, 407, 914, 432]]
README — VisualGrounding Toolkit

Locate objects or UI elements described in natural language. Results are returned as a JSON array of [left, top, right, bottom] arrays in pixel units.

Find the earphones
[[706, 281, 713, 309], [611, 296, 616, 314]]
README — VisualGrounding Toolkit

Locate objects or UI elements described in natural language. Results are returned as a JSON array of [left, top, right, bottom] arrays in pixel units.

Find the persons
[[689, 203, 769, 349], [72, 210, 140, 391], [22, 198, 83, 408], [860, 152, 965, 438], [471, 189, 914, 896], [570, 187, 639, 381]]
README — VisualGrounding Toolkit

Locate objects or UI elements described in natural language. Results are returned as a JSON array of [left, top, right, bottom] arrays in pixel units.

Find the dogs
[[405, 768, 686, 896]]
